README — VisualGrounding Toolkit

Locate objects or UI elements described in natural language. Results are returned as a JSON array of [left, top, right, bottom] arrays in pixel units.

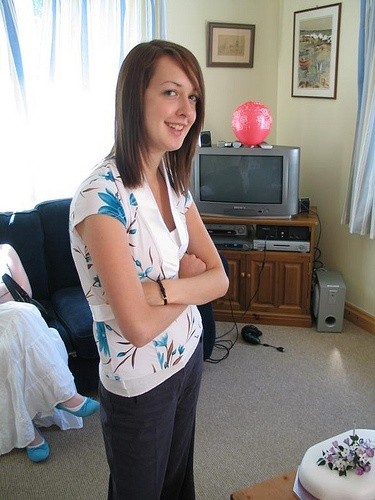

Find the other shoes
[[55, 394, 100, 418], [26, 440, 49, 463]]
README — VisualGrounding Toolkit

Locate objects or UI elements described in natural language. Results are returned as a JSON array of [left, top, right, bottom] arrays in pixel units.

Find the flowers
[[314, 420, 375, 476]]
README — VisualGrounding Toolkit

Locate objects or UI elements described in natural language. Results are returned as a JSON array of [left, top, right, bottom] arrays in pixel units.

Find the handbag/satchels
[[2, 274, 71, 353]]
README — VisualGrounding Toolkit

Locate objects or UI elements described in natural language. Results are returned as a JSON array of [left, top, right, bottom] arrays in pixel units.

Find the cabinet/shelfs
[[200, 205, 319, 328]]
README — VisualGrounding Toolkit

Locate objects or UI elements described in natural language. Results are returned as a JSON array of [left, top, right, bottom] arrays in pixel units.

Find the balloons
[[231, 101, 273, 146]]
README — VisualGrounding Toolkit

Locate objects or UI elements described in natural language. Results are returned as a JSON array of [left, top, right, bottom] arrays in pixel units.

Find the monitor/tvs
[[192, 145, 301, 219]]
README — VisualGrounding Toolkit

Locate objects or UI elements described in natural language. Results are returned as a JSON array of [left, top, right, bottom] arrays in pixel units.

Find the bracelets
[[157, 279, 167, 305]]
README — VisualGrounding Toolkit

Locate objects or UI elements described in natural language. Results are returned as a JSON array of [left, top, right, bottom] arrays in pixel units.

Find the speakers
[[298, 198, 309, 212], [200, 131, 212, 147]]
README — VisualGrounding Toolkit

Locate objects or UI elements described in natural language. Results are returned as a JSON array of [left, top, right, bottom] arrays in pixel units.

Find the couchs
[[0, 198, 229, 392]]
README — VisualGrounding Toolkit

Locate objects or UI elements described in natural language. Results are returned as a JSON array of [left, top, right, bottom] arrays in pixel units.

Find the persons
[[0, 243, 100, 462], [69, 39, 229, 500]]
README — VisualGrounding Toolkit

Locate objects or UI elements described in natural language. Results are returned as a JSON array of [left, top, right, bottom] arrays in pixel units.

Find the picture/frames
[[291, 2, 343, 99], [208, 22, 256, 69]]
